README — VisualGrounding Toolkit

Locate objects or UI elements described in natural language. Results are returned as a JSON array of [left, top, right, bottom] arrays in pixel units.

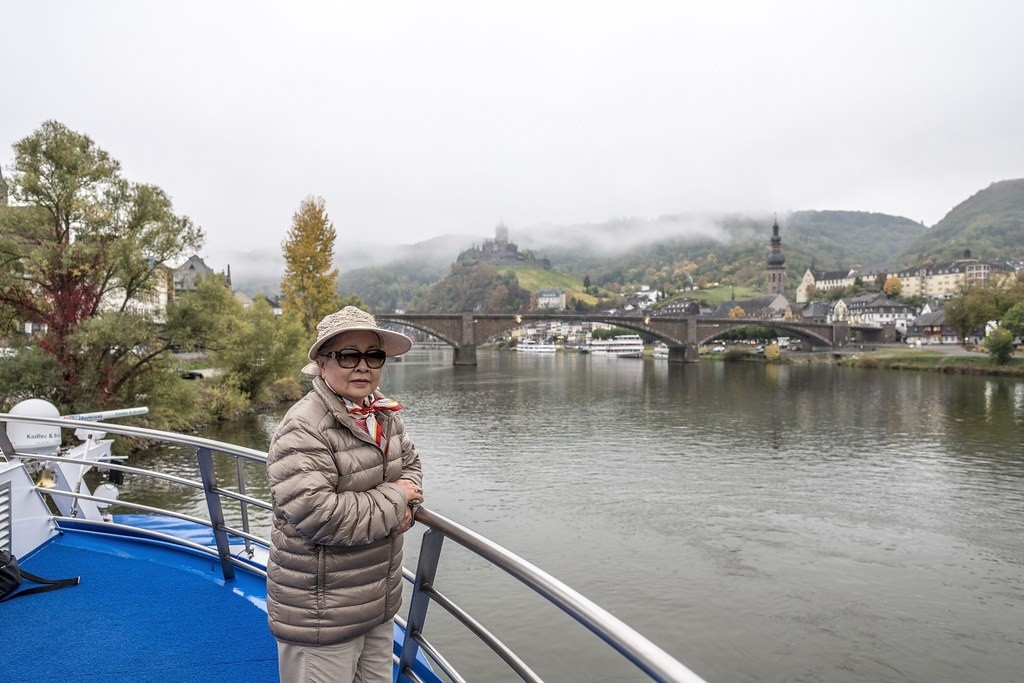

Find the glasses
[[320, 348, 386, 370]]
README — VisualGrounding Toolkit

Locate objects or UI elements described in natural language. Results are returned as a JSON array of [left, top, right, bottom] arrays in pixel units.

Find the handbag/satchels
[[0, 549, 22, 598]]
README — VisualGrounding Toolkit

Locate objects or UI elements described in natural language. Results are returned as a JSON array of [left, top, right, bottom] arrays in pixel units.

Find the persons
[[267, 306, 424, 682]]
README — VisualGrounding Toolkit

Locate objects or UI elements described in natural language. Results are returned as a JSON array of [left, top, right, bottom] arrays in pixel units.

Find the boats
[[617, 352, 641, 358], [395, 356, 402, 360], [517, 345, 556, 353]]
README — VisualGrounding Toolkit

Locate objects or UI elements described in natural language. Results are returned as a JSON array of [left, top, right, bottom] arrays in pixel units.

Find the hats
[[308, 306, 414, 362]]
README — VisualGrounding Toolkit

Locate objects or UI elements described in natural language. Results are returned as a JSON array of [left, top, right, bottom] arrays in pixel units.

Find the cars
[[757, 345, 765, 354], [713, 346, 724, 353], [787, 345, 802, 351]]
